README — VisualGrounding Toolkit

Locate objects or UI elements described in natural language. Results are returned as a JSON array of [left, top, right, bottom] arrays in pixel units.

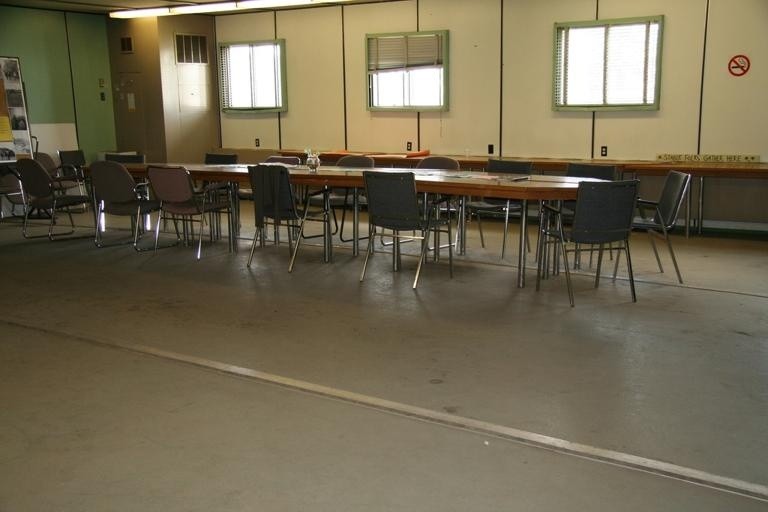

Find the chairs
[[362, 156, 693, 308], [1, 151, 375, 274]]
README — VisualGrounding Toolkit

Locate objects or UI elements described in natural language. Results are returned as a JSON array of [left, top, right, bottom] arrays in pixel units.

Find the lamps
[[106, 1, 324, 19]]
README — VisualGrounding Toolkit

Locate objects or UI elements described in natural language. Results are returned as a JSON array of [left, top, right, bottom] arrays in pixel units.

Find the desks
[[693, 159, 766, 237]]
